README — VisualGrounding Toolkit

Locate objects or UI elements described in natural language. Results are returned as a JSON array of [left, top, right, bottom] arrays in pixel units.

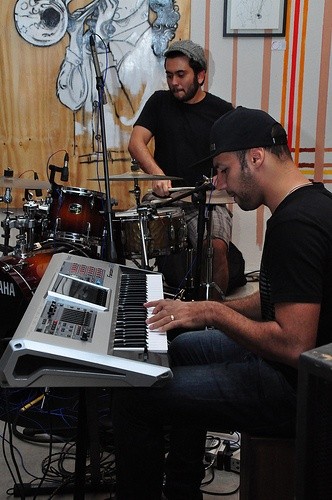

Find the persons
[[128, 40, 235, 300], [114, 106, 332, 500]]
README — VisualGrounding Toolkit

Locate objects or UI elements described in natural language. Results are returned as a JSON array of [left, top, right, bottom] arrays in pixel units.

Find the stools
[[240, 431, 297, 500]]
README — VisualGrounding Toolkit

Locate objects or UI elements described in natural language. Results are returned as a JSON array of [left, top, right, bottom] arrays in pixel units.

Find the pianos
[[0, 252, 176, 386]]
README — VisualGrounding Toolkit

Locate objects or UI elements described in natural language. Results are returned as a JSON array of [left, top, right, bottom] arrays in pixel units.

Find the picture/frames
[[223, 0, 288, 38]]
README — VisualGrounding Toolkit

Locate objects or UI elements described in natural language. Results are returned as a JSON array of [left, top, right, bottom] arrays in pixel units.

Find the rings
[[171, 315, 174, 321]]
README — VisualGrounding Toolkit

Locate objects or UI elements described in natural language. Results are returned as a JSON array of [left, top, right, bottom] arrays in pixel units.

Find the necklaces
[[276, 182, 312, 209]]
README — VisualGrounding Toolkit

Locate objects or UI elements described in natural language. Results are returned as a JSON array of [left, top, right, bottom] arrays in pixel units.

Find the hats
[[163, 39, 206, 72], [198, 105, 288, 162]]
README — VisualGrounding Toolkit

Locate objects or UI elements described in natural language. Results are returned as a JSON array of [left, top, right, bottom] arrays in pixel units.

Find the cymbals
[[0, 175, 62, 188], [172, 189, 237, 204], [88, 170, 184, 180]]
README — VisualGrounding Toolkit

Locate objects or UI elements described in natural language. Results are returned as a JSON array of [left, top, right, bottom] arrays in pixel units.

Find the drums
[[44, 185, 110, 244], [114, 207, 184, 257], [0, 240, 97, 352]]
